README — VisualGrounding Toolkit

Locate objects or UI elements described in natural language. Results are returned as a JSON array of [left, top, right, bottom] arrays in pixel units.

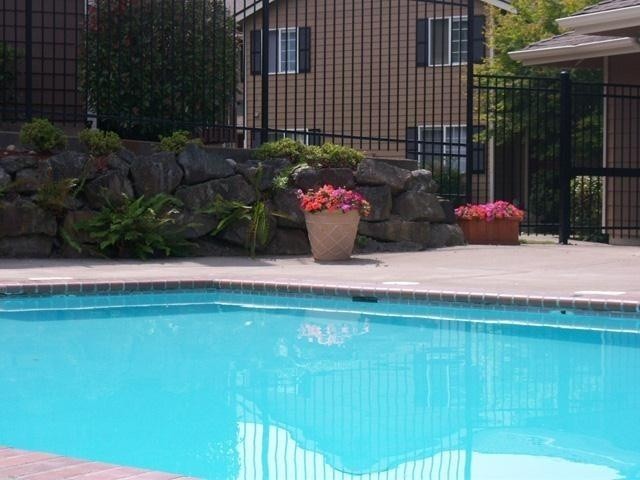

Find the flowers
[[293, 182, 372, 220], [454, 200, 526, 223]]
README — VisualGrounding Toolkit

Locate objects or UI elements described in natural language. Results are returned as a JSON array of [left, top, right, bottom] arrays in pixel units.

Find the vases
[[303, 211, 361, 261], [459, 219, 520, 246]]
